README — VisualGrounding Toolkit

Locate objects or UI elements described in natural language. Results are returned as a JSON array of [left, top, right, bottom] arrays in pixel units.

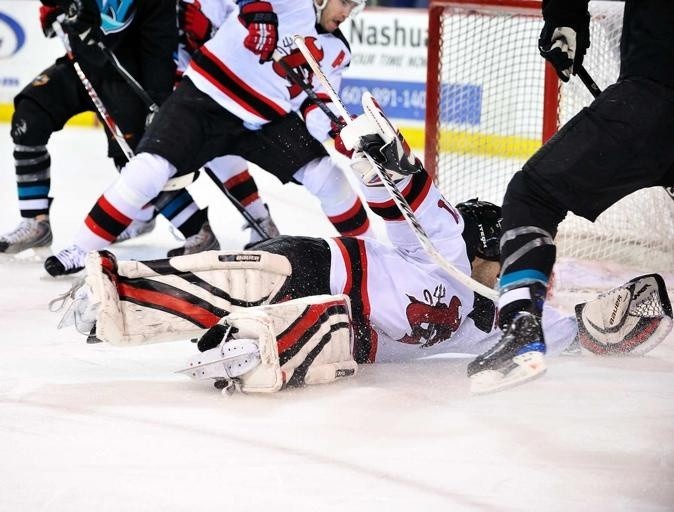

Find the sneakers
[[0, 220, 53, 254], [112, 219, 156, 242], [466, 313, 545, 376], [45, 245, 88, 277], [167, 225, 219, 257], [249, 203, 279, 244]]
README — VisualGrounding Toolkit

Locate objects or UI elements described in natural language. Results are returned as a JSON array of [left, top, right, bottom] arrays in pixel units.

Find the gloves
[[40, 0, 68, 37], [238, 11, 279, 63], [178, 1, 213, 48], [538, 1, 591, 82], [57, 1, 110, 72], [330, 114, 358, 159]]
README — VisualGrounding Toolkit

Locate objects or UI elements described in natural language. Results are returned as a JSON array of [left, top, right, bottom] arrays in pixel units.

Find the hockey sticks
[[294, 37, 499, 304], [51, 14, 199, 192]]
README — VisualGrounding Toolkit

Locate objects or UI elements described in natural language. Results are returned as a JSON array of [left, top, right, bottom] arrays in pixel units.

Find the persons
[[41, 0, 386, 280], [47, 92, 674, 394], [466, 1, 674, 393], [0, 0, 222, 262]]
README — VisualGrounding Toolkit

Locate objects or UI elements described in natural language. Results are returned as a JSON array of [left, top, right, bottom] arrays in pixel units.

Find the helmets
[[314, 0, 365, 25], [454, 198, 503, 263]]
